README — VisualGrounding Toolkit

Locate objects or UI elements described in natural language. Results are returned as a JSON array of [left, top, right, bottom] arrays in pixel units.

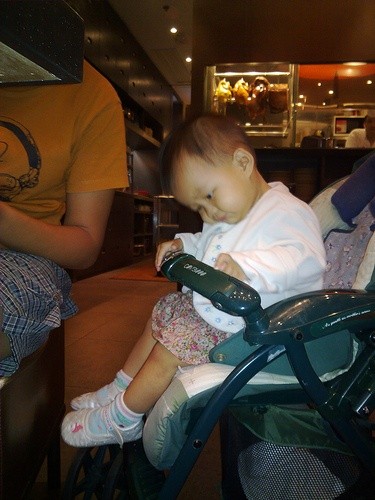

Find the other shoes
[[70, 381, 122, 411], [61, 404, 144, 447]]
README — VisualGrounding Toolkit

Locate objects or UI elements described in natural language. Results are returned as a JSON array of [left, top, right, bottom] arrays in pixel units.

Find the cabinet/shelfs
[[108, 191, 153, 270], [330, 114, 366, 149]]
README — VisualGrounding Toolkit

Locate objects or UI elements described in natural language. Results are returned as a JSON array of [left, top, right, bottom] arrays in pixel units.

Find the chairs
[[0, 266, 67, 500]]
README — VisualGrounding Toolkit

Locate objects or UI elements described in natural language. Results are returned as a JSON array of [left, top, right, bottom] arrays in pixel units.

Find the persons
[[60, 111, 325, 447], [346, 113, 375, 148], [0, 58, 130, 377]]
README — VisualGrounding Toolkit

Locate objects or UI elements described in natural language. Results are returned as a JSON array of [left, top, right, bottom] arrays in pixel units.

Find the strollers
[[60, 152, 375, 500]]
